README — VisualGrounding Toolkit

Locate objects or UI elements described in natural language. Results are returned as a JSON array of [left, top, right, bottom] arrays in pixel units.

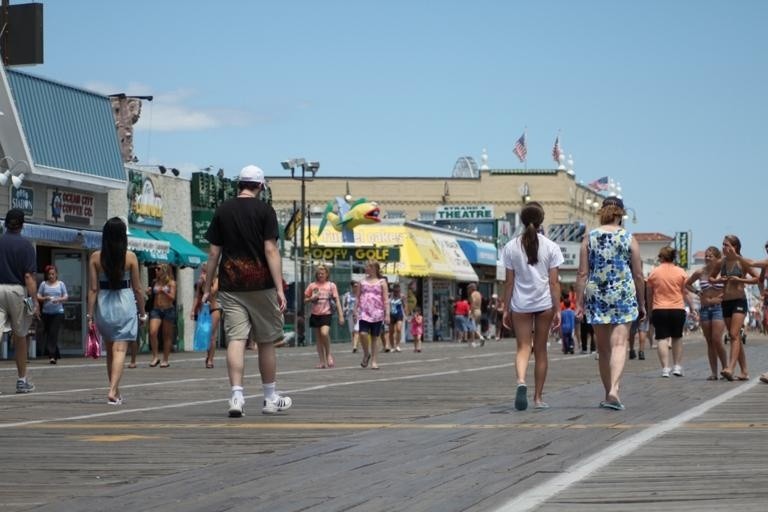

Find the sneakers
[[262, 395, 292, 414], [361, 355, 370, 367], [228, 397, 246, 417], [630, 350, 645, 359], [16, 377, 35, 393], [662, 365, 684, 377], [316, 355, 335, 368]]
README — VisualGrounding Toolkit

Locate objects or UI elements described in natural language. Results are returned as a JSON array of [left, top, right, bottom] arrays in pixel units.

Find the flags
[[552, 137, 561, 166], [512, 133, 528, 163]]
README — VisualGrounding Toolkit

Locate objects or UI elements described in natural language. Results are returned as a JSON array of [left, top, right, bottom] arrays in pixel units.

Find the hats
[[5, 208, 24, 225], [239, 165, 267, 190]]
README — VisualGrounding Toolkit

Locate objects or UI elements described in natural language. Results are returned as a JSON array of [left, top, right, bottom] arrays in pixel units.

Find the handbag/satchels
[[85, 325, 100, 358], [193, 303, 213, 351], [146, 281, 156, 312], [329, 298, 336, 313]]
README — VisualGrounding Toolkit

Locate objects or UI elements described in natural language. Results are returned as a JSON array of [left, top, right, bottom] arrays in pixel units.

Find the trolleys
[[724, 326, 748, 345]]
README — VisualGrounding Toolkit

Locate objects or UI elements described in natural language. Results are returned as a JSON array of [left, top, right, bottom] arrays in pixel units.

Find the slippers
[[515, 384, 527, 410], [599, 400, 624, 410], [107, 394, 125, 405], [535, 402, 550, 409], [707, 369, 768, 383]]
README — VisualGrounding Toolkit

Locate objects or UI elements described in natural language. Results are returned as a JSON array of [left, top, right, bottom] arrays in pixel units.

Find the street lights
[[0, 157, 32, 192], [281, 155, 320, 346]]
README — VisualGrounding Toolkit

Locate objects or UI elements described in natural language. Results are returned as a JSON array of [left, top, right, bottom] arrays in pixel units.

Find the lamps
[[134, 162, 167, 175], [572, 189, 637, 225], [518, 181, 531, 206], [165, 166, 181, 176], [343, 178, 352, 202], [8, 157, 28, 189], [440, 178, 452, 205], [0, 154, 15, 185]]
